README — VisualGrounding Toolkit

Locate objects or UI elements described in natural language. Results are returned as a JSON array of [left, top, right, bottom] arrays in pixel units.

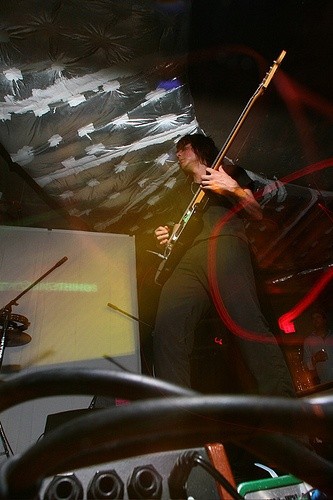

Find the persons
[[153, 131, 297, 400]]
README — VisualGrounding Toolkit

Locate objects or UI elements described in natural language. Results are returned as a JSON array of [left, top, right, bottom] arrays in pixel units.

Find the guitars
[[153, 49, 289, 287]]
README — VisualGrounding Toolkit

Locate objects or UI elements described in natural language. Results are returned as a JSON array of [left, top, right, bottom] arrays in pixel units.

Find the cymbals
[[0, 329, 32, 347]]
